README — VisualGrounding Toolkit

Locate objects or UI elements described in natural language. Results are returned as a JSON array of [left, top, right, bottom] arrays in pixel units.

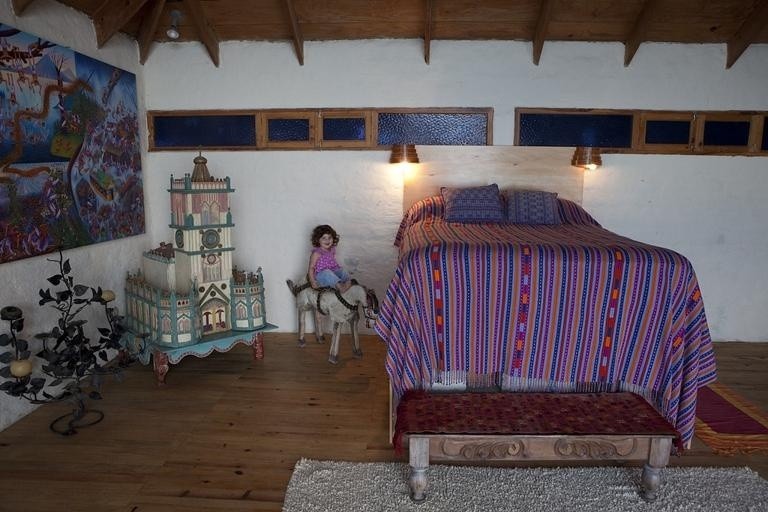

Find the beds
[[377, 189, 719, 466]]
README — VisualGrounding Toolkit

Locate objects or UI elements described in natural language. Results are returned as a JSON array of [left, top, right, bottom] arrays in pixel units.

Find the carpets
[[689, 378, 767, 459], [280, 453, 766, 512]]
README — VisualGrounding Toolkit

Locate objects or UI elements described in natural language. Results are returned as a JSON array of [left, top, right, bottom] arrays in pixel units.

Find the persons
[[308, 225, 351, 293]]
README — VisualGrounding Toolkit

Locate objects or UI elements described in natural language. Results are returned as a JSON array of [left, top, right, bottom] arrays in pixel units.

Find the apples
[[101, 290, 115, 301], [10, 359, 32, 376]]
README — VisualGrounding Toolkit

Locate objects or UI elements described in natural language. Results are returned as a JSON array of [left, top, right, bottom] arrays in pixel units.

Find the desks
[[115, 316, 279, 387]]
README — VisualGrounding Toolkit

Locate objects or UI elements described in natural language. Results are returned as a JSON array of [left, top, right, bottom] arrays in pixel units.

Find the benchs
[[391, 390, 682, 503]]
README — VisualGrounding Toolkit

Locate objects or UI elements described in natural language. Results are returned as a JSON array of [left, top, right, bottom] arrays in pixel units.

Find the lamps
[[162, 7, 181, 40], [570, 145, 603, 171], [388, 142, 420, 166]]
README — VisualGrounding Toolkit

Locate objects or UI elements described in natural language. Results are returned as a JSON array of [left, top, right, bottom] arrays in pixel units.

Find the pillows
[[506, 188, 565, 223], [439, 181, 506, 225]]
